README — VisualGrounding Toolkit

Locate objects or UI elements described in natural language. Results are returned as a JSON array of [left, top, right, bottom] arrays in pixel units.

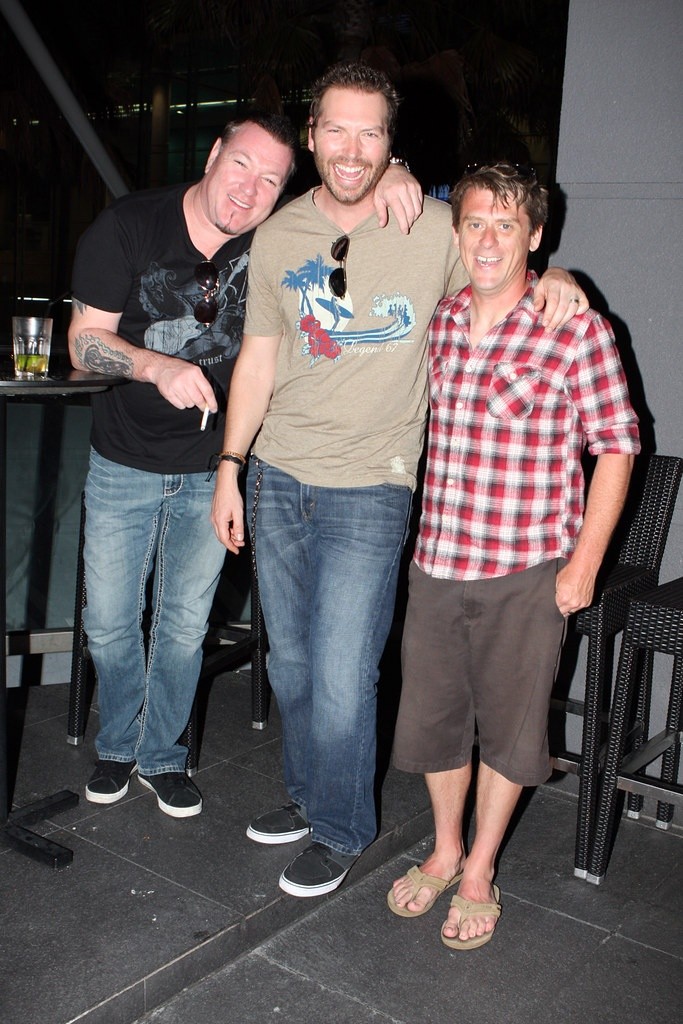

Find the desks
[[0, 366, 132, 870]]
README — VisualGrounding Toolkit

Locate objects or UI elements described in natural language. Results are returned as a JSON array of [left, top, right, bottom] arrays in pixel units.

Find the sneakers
[[247, 800, 313, 845], [279, 842, 359, 897], [137, 772, 203, 818], [85, 759, 138, 804]]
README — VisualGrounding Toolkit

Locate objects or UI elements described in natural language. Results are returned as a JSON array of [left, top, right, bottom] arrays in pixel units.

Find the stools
[[65, 487, 272, 775], [550, 450, 683, 884]]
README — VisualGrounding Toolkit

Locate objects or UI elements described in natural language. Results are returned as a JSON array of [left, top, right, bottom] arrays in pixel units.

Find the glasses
[[194, 261, 218, 323], [328, 235, 350, 300], [464, 160, 534, 176]]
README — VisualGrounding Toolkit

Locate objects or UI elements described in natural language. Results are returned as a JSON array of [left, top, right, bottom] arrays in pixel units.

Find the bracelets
[[390, 156, 412, 174], [204, 451, 246, 482]]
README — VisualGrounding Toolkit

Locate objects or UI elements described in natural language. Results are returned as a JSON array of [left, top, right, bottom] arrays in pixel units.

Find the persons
[[67, 111, 424, 817], [209, 63, 590, 899], [384, 159, 642, 951]]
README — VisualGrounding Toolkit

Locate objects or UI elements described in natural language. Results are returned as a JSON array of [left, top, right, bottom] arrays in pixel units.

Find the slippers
[[441, 885, 501, 950], [387, 864, 463, 918]]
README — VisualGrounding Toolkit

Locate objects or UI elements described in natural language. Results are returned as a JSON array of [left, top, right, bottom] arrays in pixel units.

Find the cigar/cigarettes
[[200, 402, 208, 431]]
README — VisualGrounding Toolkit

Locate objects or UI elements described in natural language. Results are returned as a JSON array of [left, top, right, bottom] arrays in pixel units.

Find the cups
[[12, 317, 53, 380]]
